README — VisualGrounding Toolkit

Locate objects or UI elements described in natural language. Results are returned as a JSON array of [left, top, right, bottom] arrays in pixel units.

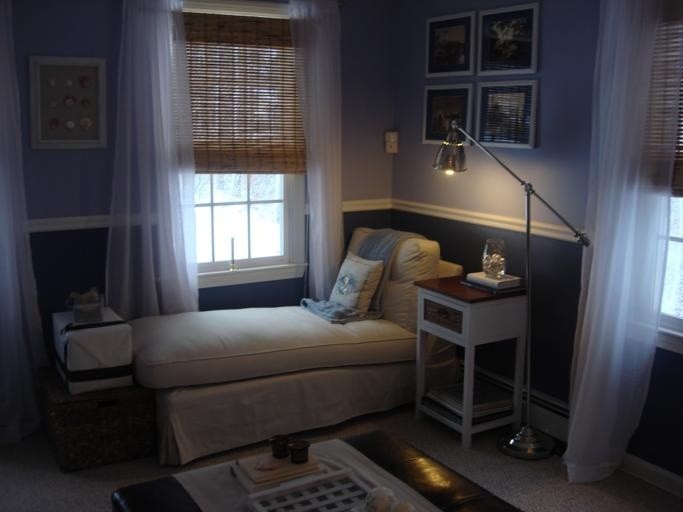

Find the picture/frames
[[420, 4, 541, 151], [29, 56, 108, 152]]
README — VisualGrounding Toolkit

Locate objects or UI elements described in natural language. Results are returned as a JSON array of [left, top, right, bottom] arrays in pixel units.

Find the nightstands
[[411, 274, 527, 451]]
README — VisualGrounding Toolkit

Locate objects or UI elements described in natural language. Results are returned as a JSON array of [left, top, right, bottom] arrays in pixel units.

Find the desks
[[109, 431, 520, 511]]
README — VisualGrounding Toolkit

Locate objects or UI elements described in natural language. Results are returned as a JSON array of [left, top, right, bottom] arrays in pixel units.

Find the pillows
[[327, 224, 441, 335]]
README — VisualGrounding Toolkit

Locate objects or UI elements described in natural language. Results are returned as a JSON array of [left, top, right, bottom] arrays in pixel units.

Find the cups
[[288, 440, 311, 465], [269, 434, 291, 459]]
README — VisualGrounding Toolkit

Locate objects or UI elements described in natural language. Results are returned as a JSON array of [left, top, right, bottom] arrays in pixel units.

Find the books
[[236, 453, 321, 483], [458, 278, 524, 296], [466, 270, 521, 289], [228, 458, 351, 502]]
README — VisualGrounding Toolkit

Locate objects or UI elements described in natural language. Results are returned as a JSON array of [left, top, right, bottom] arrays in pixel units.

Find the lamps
[[437, 102, 590, 461]]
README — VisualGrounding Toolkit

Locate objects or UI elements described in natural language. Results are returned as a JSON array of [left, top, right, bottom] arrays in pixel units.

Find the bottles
[[481, 238, 506, 279]]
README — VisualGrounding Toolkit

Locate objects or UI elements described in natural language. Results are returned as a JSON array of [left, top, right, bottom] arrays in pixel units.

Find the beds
[[126, 258, 464, 465]]
